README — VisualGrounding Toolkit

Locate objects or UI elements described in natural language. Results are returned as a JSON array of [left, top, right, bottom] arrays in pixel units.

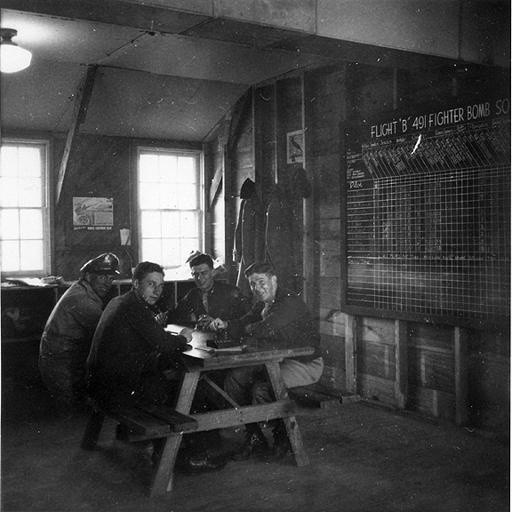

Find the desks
[[82, 322, 317, 499]]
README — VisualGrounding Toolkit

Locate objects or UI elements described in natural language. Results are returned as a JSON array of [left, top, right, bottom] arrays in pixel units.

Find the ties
[[261, 302, 270, 320], [202, 291, 208, 313]]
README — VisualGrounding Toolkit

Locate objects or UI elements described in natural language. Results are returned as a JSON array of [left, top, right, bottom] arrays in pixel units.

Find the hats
[[80, 253, 121, 276], [240, 177, 255, 199]]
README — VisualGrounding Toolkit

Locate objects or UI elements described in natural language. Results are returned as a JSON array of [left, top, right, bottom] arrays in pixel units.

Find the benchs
[[84, 380, 199, 443], [291, 374, 362, 413]]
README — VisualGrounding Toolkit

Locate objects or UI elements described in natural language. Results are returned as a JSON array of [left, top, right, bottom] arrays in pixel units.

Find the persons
[[38, 252, 122, 392], [210, 263, 324, 460], [85, 261, 228, 475], [154, 252, 250, 332]]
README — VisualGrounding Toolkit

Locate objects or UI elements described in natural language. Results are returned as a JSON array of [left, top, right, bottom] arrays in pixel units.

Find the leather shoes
[[115, 422, 226, 472]]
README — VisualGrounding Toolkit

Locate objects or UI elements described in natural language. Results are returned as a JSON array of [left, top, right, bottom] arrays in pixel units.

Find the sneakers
[[266, 432, 289, 465], [232, 430, 266, 462]]
